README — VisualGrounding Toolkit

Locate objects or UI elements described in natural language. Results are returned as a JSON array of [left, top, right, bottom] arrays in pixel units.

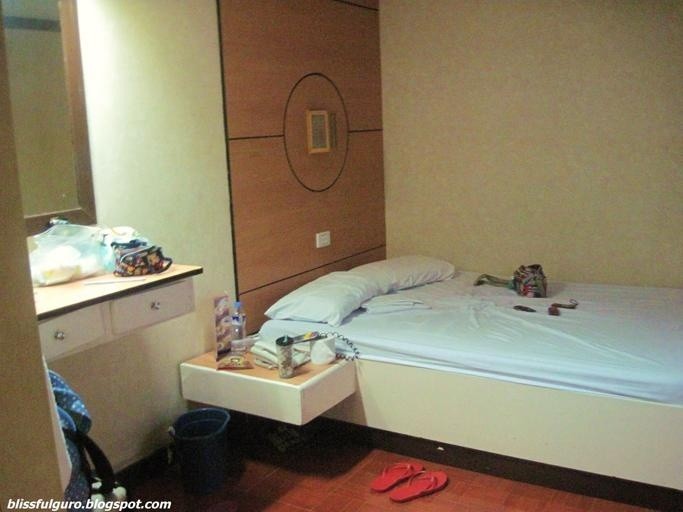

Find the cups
[[274, 336, 294, 378]]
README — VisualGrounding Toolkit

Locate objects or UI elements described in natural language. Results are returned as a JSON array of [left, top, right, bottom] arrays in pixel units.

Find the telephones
[[251, 339, 310, 369]]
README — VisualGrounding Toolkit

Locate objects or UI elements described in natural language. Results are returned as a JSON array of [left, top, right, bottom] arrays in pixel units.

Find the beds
[[239, 273, 683, 493]]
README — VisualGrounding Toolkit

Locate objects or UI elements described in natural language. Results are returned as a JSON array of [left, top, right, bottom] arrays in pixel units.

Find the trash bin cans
[[168, 408, 232, 495]]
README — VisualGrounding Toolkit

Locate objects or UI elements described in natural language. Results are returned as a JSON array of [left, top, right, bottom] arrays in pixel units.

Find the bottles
[[227, 301, 247, 354]]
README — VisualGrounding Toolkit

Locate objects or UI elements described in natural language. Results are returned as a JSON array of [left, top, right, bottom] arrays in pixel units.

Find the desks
[[33, 260, 204, 363]]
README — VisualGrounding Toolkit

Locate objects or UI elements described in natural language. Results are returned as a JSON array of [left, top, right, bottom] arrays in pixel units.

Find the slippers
[[388, 468, 448, 503], [370, 460, 424, 493]]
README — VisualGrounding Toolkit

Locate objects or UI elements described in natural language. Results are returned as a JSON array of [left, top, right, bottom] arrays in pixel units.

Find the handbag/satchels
[[511, 262, 549, 299], [113, 241, 173, 279]]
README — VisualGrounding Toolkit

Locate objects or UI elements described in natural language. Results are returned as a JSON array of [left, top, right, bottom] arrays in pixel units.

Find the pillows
[[262, 255, 455, 328]]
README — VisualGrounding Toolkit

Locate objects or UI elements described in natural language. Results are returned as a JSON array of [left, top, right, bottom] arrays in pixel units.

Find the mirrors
[[0, 1, 99, 238]]
